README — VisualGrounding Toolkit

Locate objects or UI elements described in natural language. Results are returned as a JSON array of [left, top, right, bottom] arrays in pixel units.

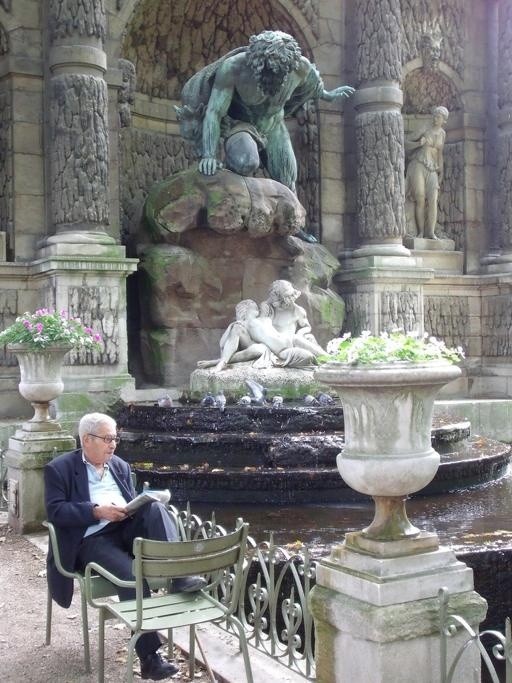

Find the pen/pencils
[[112, 503, 133, 520]]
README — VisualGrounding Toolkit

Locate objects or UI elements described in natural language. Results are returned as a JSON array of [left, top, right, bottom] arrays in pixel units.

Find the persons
[[404, 104, 451, 241], [219, 296, 332, 359], [196, 278, 312, 373], [197, 29, 357, 243], [40, 411, 209, 682]]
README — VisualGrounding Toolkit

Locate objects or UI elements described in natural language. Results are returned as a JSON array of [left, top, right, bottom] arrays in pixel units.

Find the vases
[[315, 367, 462, 540], [8, 345, 75, 423]]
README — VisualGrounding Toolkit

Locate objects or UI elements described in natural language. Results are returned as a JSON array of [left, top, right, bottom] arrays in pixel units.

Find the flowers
[[1, 310, 105, 354], [317, 325, 465, 364]]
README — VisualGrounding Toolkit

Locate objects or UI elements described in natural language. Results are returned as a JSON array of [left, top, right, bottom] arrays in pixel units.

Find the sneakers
[[169, 575, 207, 593], [140, 653, 179, 680]]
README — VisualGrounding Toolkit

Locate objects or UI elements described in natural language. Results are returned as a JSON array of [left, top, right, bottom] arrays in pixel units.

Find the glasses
[[88, 433, 121, 444]]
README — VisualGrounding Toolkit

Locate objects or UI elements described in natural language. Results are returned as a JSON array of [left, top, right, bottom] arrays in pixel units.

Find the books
[[84, 486, 171, 538]]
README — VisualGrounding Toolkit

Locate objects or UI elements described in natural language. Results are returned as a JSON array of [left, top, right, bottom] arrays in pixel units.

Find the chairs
[[42, 514, 121, 672], [83, 513, 258, 680]]
